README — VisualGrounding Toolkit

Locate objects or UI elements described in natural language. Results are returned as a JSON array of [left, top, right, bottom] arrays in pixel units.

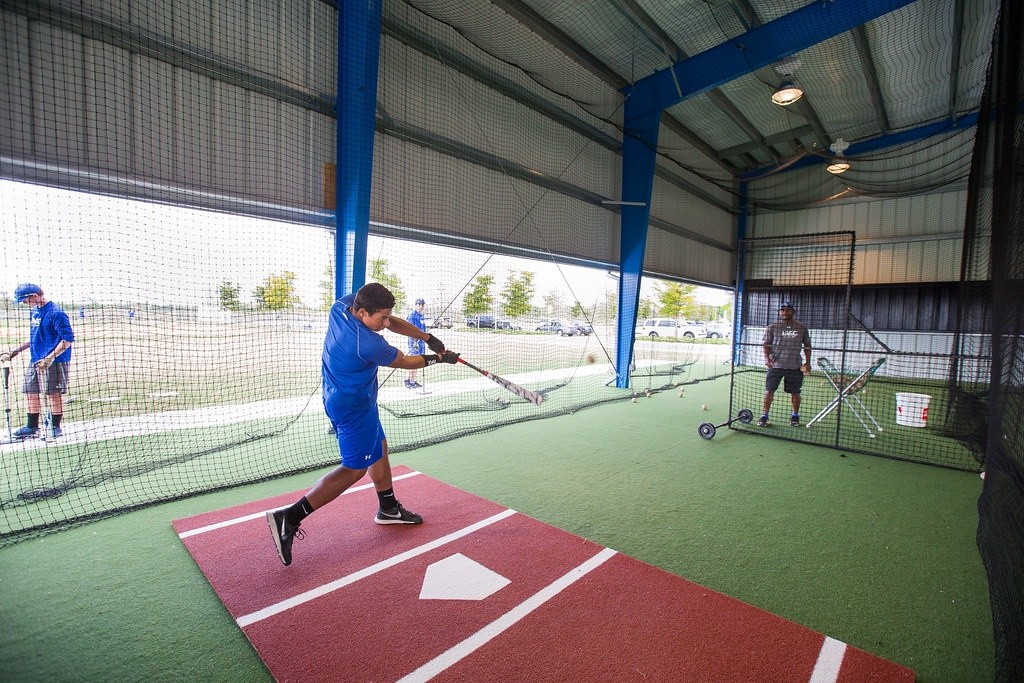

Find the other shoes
[[405, 380, 422, 389]]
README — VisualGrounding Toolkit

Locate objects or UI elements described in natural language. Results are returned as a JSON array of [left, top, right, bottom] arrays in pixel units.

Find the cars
[[535, 319, 578, 336], [570, 320, 594, 336]]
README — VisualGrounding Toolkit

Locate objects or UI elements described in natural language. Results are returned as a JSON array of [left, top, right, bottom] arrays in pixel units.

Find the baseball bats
[[428, 346, 543, 405]]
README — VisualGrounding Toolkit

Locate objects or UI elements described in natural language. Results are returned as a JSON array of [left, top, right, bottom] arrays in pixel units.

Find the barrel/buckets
[[896, 393, 932, 428]]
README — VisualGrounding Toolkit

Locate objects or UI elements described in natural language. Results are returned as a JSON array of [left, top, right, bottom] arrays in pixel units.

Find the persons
[[404, 299, 426, 389], [266, 282, 461, 566], [757, 302, 812, 426], [0, 283, 74, 437], [76, 304, 84, 323], [129, 310, 135, 323]]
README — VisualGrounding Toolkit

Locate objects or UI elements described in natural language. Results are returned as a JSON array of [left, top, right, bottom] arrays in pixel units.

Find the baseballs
[[646, 393, 650, 397], [674, 382, 678, 387], [2, 354, 10, 361], [632, 398, 636, 402], [681, 387, 685, 391], [679, 393, 683, 398], [702, 404, 708, 410]]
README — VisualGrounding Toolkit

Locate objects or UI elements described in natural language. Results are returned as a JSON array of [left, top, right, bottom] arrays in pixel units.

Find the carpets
[[172, 464, 915, 683]]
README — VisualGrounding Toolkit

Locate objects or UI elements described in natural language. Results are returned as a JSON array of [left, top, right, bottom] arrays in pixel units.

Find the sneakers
[[374, 502, 423, 525], [791, 418, 800, 427], [12, 426, 41, 438], [41, 423, 62, 442], [757, 416, 770, 427], [267, 510, 308, 565]]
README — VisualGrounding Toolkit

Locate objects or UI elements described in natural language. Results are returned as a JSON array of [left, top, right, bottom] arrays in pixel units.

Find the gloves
[[426, 332, 445, 356], [440, 352, 460, 364]]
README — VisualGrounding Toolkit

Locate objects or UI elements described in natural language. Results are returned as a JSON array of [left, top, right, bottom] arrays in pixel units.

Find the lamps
[[826, 139, 851, 174], [770, 57, 804, 106]]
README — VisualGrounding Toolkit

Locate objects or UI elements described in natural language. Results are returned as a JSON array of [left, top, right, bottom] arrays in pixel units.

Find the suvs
[[423, 317, 454, 329], [467, 315, 521, 332], [641, 318, 731, 340]]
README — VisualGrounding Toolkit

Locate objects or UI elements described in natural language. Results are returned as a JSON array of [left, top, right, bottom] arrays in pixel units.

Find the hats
[[778, 302, 795, 311], [13, 284, 40, 304], [416, 298, 426, 304]]
